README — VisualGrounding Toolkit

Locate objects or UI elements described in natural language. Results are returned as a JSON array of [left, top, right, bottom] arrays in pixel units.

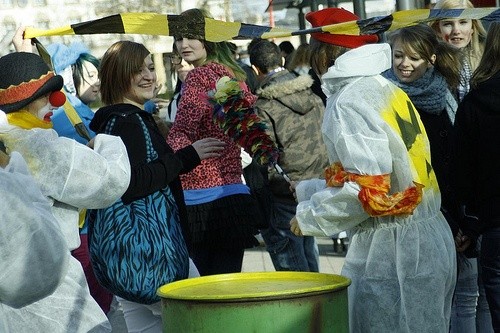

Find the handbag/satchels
[[86, 112, 188, 304]]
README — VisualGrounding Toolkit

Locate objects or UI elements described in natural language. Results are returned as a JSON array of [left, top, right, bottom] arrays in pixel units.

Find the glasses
[[170, 53, 182, 64]]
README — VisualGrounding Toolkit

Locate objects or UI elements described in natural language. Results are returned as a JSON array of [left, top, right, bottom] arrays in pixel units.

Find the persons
[[94, 40, 227, 333], [454, 8, 500, 333], [428, 1, 487, 101], [246, 37, 334, 272], [164, 8, 258, 277], [381, 23, 494, 333], [225, 38, 267, 96], [44, 40, 113, 317], [294, 44, 327, 107], [278, 40, 295, 71], [166, 43, 196, 122], [0, 51, 129, 333], [298, 7, 459, 333]]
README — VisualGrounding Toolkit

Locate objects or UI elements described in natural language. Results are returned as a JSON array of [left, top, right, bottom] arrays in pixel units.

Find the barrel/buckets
[[157, 271, 351, 333]]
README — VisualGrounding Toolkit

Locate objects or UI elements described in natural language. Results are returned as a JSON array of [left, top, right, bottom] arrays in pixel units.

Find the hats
[[0, 52, 63, 113], [305, 8, 379, 49]]
